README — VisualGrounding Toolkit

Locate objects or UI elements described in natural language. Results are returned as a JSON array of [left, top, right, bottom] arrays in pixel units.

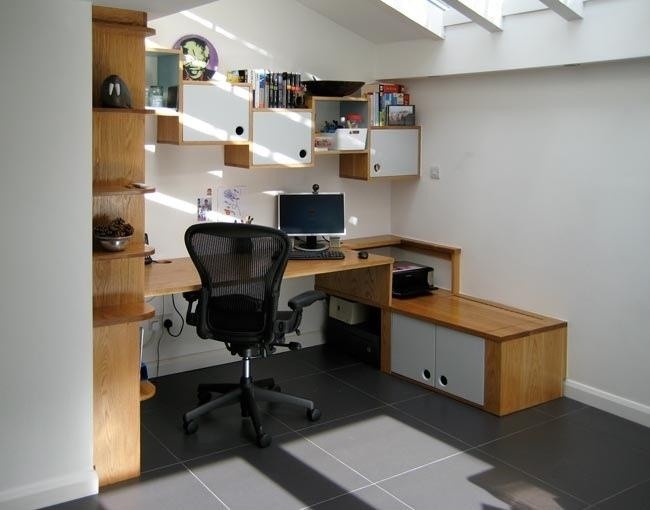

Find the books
[[225, 68, 305, 109], [367, 83, 416, 127]]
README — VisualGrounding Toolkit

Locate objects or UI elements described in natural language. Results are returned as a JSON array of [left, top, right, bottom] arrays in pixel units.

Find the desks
[[142, 235, 394, 378]]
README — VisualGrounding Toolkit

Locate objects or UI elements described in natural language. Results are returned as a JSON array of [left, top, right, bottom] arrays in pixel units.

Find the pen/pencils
[[235, 216, 253, 224]]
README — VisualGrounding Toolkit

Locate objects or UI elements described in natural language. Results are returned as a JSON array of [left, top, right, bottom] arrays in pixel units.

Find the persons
[[180, 38, 216, 81], [198, 188, 213, 221]]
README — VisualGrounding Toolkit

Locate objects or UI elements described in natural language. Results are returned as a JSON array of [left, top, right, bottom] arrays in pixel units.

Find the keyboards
[[287, 250, 345, 260]]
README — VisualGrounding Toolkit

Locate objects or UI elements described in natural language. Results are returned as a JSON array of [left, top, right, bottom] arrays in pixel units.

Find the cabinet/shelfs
[[144, 47, 421, 182], [92, 20, 156, 331], [382, 292, 567, 417]]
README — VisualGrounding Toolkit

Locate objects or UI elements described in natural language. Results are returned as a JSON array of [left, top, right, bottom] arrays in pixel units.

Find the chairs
[[182, 221, 327, 450]]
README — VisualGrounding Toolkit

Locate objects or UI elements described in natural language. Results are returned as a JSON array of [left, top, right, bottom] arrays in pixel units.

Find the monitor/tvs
[[277, 191, 347, 251]]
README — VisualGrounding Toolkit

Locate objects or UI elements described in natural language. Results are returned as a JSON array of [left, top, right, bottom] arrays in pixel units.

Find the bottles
[[148, 84, 163, 108]]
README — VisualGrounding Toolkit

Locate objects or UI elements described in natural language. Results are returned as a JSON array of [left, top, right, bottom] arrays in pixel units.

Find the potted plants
[[94, 217, 135, 251]]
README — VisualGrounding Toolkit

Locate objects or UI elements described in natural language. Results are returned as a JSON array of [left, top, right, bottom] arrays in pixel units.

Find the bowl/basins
[[96, 234, 135, 252]]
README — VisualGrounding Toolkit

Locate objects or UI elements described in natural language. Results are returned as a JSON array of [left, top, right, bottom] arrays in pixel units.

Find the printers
[[391, 262, 439, 300]]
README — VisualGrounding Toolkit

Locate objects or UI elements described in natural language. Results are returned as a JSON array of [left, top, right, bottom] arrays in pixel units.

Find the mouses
[[358, 250, 368, 259]]
